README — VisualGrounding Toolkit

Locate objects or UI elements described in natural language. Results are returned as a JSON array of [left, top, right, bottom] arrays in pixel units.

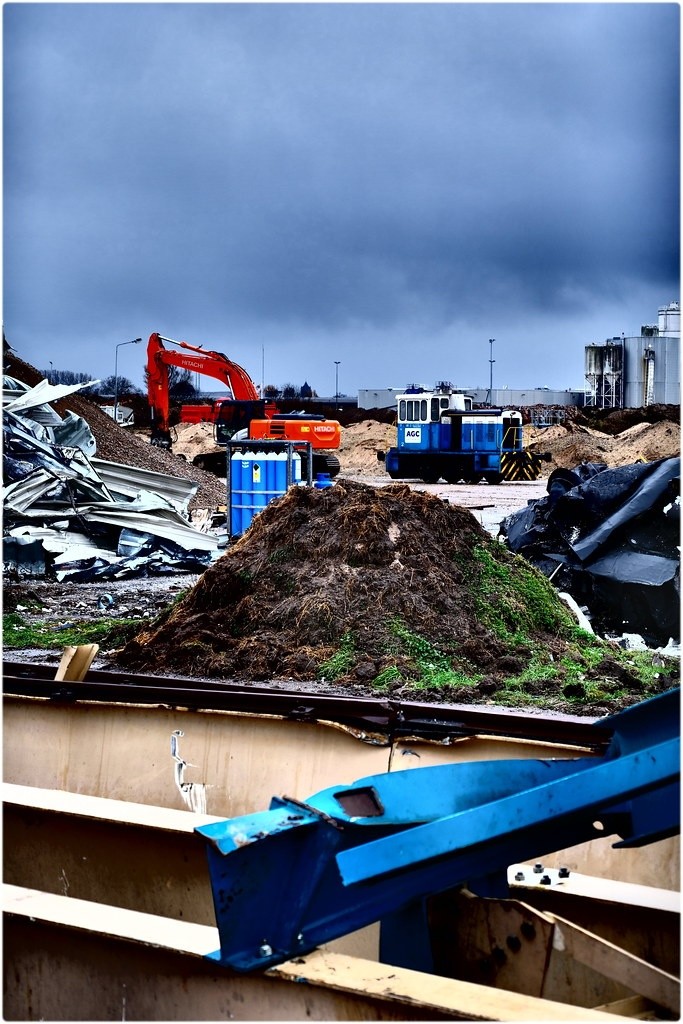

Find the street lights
[[487, 338, 496, 407], [114, 336, 142, 422], [334, 361, 341, 415]]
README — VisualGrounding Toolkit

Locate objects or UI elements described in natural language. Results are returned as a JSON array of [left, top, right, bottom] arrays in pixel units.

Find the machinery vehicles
[[376, 379, 552, 486], [142, 331, 342, 478]]
